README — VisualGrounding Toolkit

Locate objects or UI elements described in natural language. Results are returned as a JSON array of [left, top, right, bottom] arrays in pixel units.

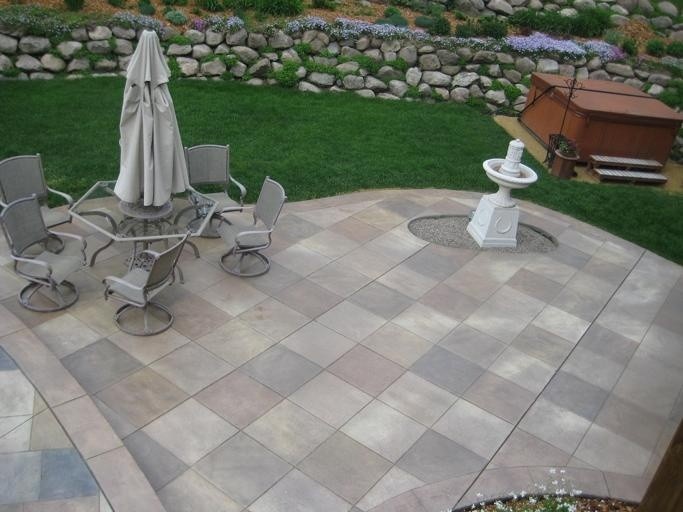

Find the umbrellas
[[111, 25, 191, 208]]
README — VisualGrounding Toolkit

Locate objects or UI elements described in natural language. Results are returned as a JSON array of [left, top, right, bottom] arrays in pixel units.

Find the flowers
[[552, 136, 581, 157]]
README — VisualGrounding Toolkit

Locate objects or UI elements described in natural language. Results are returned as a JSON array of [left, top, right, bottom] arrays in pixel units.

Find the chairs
[[216, 175, 287, 278], [102, 231, 191, 336], [184, 144, 248, 238], [0, 153, 73, 259], [0, 194, 87, 312]]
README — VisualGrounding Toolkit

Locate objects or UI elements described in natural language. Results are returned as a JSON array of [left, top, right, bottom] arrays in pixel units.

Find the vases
[[553, 153, 575, 180]]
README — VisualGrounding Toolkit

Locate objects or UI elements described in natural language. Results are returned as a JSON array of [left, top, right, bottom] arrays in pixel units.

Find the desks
[[68, 181, 220, 285]]
